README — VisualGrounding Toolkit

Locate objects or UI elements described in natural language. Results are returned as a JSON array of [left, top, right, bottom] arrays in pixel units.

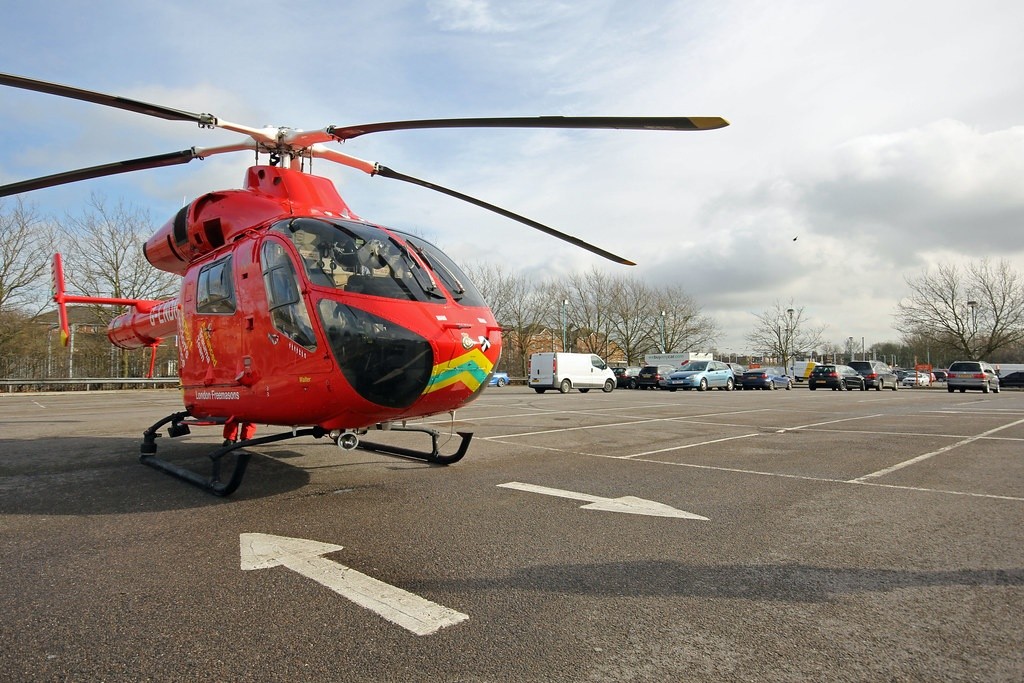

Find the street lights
[[787, 308, 794, 361], [849, 337, 854, 362], [562, 300, 569, 353], [661, 310, 666, 354], [966, 301, 977, 361]]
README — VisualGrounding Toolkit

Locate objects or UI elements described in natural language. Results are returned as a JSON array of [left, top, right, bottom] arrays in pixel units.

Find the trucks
[[526, 352, 618, 393], [790, 361, 825, 382]]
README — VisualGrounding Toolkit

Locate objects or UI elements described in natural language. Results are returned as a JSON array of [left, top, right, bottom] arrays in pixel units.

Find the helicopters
[[0, 69, 731, 500]]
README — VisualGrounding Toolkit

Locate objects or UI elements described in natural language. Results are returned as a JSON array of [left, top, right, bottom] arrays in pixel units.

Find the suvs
[[946, 360, 1000, 393], [637, 365, 679, 392], [846, 360, 900, 391], [610, 366, 643, 390]]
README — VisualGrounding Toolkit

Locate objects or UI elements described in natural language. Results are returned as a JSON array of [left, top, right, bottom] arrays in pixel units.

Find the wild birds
[[792, 236, 798, 241]]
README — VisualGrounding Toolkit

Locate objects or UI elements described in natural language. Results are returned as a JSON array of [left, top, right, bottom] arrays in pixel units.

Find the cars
[[742, 366, 794, 390], [666, 359, 735, 392], [891, 367, 948, 387], [808, 364, 867, 391], [486, 371, 510, 388], [998, 372, 1024, 388], [725, 362, 745, 391]]
